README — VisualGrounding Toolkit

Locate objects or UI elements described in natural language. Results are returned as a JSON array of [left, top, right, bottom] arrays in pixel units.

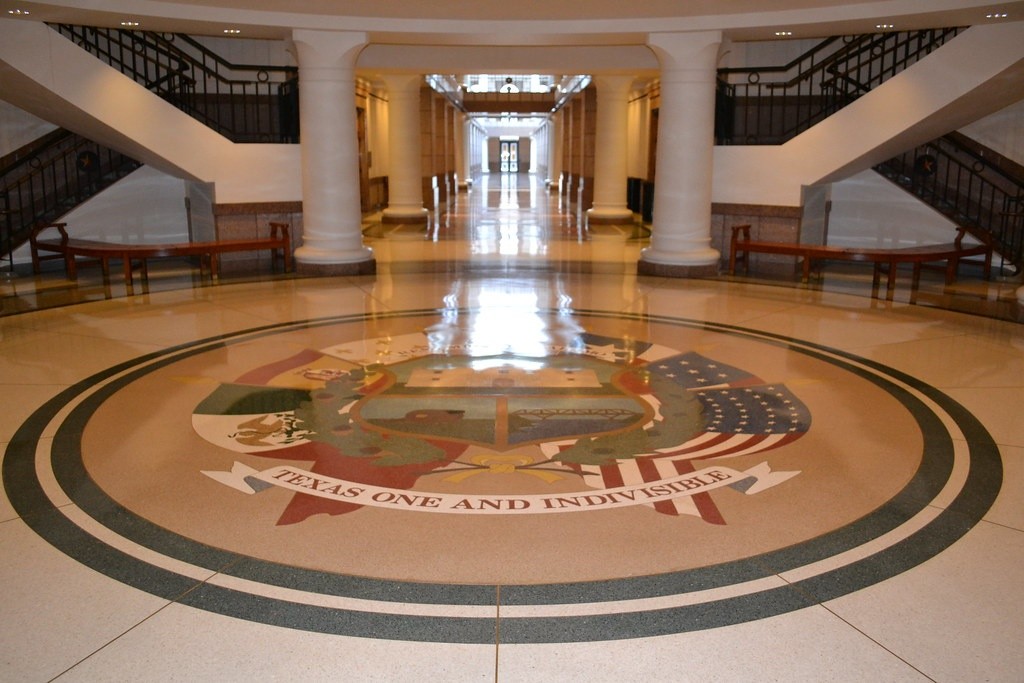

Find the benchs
[[729, 224, 998, 291], [28, 220, 294, 286]]
